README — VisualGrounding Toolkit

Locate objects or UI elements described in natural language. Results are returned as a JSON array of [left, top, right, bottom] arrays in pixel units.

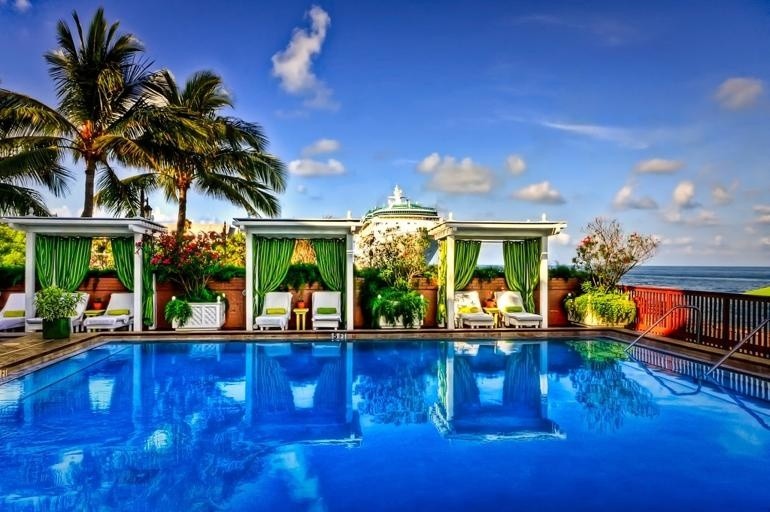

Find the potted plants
[[367, 271, 428, 329], [93, 297, 104, 309], [296, 296, 304, 307], [33, 286, 83, 339]]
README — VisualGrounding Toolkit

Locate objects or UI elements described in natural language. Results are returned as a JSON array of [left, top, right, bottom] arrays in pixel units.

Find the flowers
[[133, 230, 234, 301], [570, 219, 659, 323]]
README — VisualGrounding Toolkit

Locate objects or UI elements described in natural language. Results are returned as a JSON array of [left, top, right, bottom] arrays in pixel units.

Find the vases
[[166, 293, 226, 332], [562, 301, 630, 328]]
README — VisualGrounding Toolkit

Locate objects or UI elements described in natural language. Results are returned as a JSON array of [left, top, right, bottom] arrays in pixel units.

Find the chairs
[[311, 291, 342, 330], [454, 289, 494, 329], [255, 292, 292, 330], [0, 293, 36, 332], [494, 290, 543, 329], [26, 293, 90, 334], [83, 293, 133, 332]]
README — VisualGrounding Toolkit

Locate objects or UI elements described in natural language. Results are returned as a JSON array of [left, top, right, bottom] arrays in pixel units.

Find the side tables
[[293, 307, 309, 330], [84, 310, 104, 332], [484, 306, 499, 328]]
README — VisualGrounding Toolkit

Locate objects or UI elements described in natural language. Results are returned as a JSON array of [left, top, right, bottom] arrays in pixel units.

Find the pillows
[[459, 306, 479, 313], [506, 306, 522, 313], [317, 308, 336, 314], [266, 308, 284, 314], [108, 310, 129, 315], [3, 311, 25, 317]]
